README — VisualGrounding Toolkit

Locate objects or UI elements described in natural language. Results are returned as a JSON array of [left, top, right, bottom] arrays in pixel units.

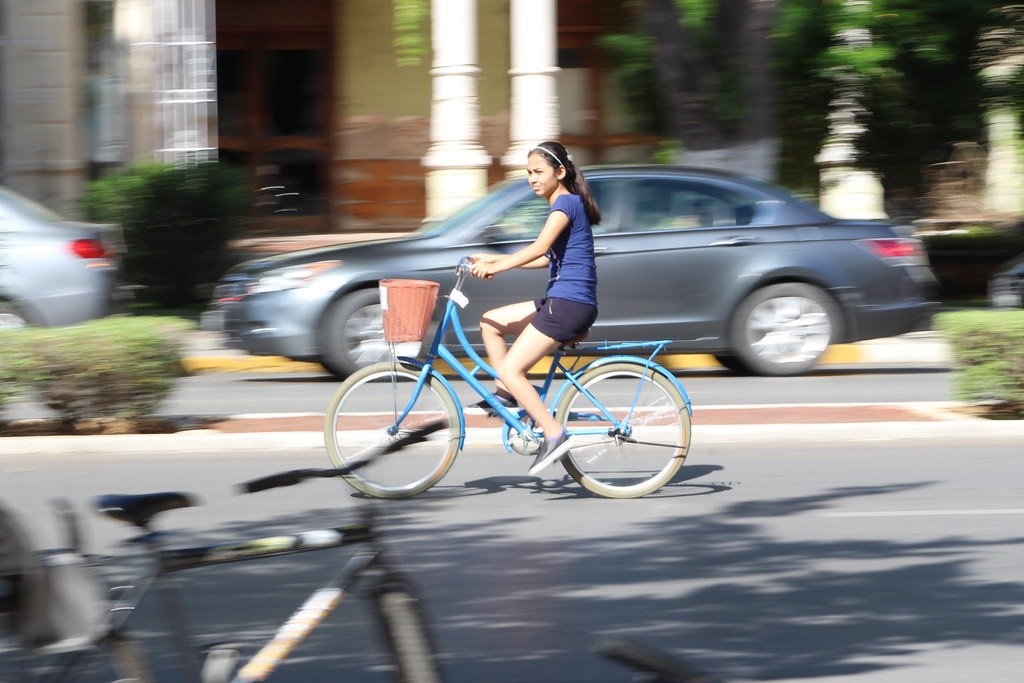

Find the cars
[[206, 166, 940, 376], [0, 189, 128, 332]]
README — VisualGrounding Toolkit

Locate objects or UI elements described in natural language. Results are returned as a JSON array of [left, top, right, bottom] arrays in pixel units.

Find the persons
[[463, 139, 599, 473]]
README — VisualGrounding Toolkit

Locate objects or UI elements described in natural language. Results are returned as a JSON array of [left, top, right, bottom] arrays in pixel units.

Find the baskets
[[378, 279, 441, 344]]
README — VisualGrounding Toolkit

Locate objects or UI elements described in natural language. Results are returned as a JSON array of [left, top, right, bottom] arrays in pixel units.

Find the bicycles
[[326, 255, 694, 502], [0, 419, 445, 683]]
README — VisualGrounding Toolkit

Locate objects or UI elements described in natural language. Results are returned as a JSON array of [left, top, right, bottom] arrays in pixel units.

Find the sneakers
[[527, 428, 576, 476], [461, 391, 522, 416]]
